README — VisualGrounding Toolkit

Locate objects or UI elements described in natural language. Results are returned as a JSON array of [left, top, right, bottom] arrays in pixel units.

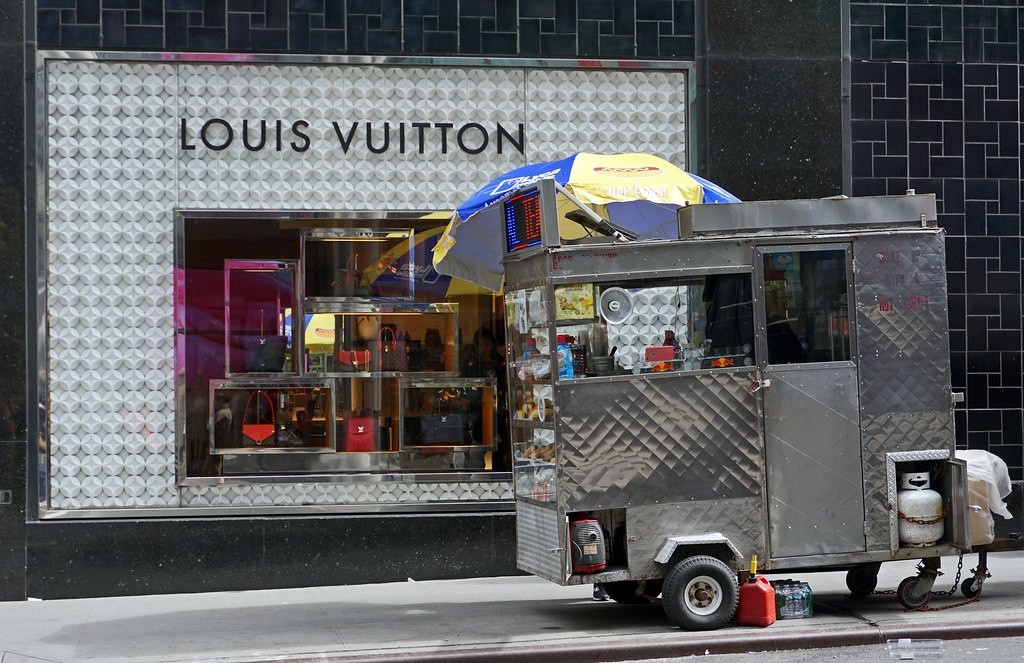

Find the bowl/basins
[[591, 356, 614, 372]]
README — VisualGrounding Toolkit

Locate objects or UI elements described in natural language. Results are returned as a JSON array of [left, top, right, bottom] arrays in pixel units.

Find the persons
[[464, 328, 506, 466]]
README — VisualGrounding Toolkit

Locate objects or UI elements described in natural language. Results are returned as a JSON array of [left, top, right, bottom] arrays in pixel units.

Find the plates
[[583, 372, 617, 377]]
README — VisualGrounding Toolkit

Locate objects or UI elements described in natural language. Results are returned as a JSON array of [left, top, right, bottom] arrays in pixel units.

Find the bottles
[[736, 576, 776, 628], [522, 337, 540, 361], [556, 335, 575, 379], [769, 578, 814, 620]]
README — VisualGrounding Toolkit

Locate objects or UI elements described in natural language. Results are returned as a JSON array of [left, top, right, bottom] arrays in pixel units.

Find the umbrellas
[[432, 151, 742, 293], [359, 224, 496, 294]]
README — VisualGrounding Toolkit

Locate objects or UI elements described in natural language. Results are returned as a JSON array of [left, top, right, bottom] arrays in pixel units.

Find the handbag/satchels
[[421, 414, 465, 445], [239, 334, 288, 372], [241, 391, 276, 447], [338, 327, 409, 372], [276, 421, 310, 447], [343, 407, 377, 451]]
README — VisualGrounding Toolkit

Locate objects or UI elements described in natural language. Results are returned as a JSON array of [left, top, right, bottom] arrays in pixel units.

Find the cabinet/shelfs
[[208, 227, 498, 476]]
